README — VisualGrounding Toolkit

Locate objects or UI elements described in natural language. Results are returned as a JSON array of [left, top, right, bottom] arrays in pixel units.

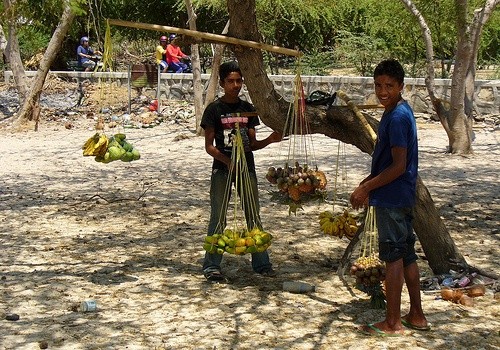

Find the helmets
[[80, 36, 90, 45], [160, 36, 168, 41], [169, 34, 178, 41]]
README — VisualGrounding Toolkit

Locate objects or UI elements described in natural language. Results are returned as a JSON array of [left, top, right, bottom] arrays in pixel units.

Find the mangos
[[203, 228, 274, 254], [94, 134, 140, 163]]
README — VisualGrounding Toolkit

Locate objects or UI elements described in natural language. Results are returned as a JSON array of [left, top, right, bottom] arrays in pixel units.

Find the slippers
[[358, 323, 403, 337], [400, 317, 431, 331]]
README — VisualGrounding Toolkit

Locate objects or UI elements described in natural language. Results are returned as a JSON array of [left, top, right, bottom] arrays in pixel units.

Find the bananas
[[82, 132, 109, 156], [318, 210, 360, 239]]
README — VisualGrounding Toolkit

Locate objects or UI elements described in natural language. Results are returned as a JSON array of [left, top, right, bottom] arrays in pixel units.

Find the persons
[[199, 62, 289, 281], [348, 59, 432, 335], [76, 37, 102, 72], [166, 34, 190, 74], [155, 36, 170, 73]]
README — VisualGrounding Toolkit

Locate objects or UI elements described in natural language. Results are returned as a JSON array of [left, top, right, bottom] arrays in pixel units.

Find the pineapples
[[288, 170, 328, 201]]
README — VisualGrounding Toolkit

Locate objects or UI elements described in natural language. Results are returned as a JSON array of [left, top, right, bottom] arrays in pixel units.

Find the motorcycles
[[157, 56, 192, 73], [66, 53, 110, 72]]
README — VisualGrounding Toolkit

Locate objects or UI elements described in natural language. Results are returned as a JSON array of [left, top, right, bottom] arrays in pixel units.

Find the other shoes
[[203, 264, 224, 281], [260, 268, 276, 277]]
